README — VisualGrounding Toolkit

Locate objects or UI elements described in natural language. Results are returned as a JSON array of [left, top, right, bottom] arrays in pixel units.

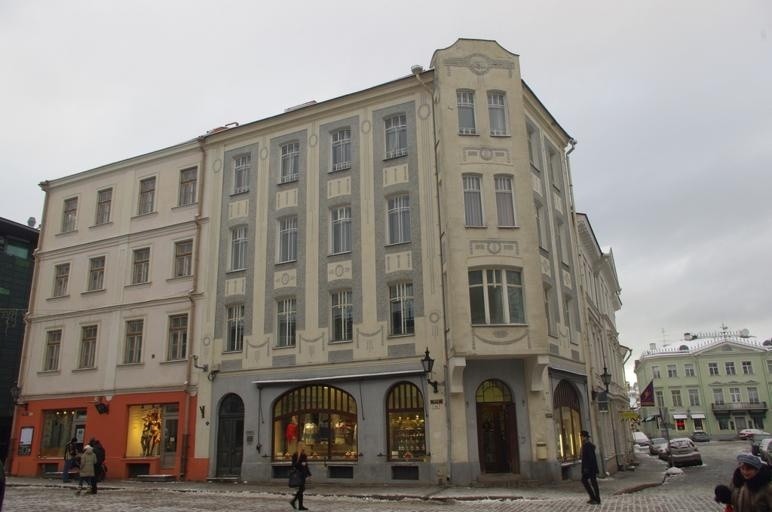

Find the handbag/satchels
[[287, 473, 303, 487]]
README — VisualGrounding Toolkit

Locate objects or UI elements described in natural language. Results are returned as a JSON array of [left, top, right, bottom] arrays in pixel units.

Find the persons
[[289, 442, 309, 510], [63, 437, 78, 482], [285, 415, 299, 458], [715, 453, 772, 512], [140, 407, 161, 455], [90, 437, 103, 482], [316, 414, 334, 459], [76, 445, 98, 494], [96, 440, 105, 480], [580, 429, 600, 504], [302, 413, 320, 459]]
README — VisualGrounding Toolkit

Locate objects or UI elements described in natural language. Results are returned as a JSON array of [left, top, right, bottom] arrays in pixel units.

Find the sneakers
[[62, 477, 97, 496], [290, 499, 309, 510], [587, 496, 601, 504]]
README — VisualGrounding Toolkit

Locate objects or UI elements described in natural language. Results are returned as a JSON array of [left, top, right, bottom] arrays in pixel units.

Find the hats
[[83, 445, 93, 450], [580, 431, 590, 437], [735, 452, 763, 470]]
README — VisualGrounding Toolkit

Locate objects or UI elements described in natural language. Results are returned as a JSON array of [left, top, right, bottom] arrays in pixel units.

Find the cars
[[632, 432, 649, 445], [649, 437, 668, 455], [739, 428, 771, 440], [691, 430, 709, 441], [666, 438, 703, 467]]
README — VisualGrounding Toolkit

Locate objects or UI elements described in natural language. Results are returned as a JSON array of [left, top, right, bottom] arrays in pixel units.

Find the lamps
[[421, 345, 438, 393], [10, 381, 27, 410], [592, 367, 611, 400]]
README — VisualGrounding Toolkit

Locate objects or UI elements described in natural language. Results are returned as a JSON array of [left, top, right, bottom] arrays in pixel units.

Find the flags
[[640, 380, 655, 406]]
